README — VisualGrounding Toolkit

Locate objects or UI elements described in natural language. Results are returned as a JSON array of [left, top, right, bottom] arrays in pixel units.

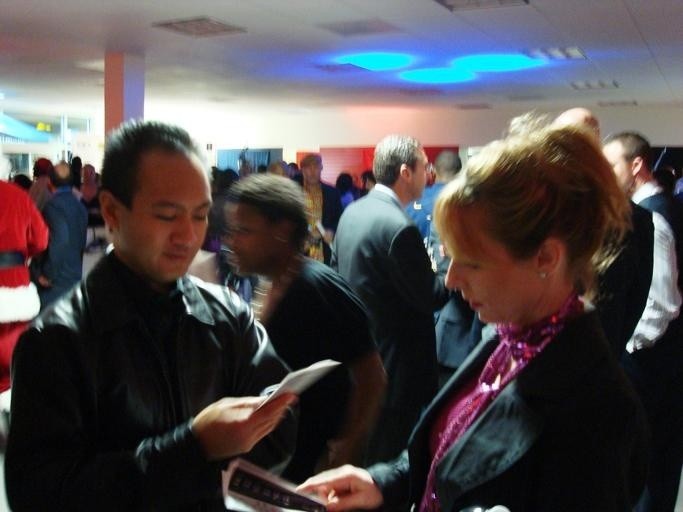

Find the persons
[[10, 174, 32, 193], [335, 173, 354, 200], [80, 164, 108, 253], [28, 158, 53, 209], [239, 160, 304, 185], [295, 154, 341, 266], [293, 113, 649, 512], [360, 171, 376, 198], [219, 172, 387, 512], [404, 151, 462, 327], [556, 107, 601, 142], [5, 121, 297, 512], [652, 168, 676, 197], [209, 166, 239, 200], [589, 131, 683, 440], [29, 162, 89, 312], [331, 134, 460, 466]]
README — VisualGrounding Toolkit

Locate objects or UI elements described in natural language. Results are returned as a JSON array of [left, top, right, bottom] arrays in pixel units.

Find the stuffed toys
[[0, 139, 48, 426]]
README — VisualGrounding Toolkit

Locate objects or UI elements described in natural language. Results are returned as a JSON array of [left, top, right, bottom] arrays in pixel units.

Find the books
[[222, 457, 327, 512], [253, 358, 341, 412]]
